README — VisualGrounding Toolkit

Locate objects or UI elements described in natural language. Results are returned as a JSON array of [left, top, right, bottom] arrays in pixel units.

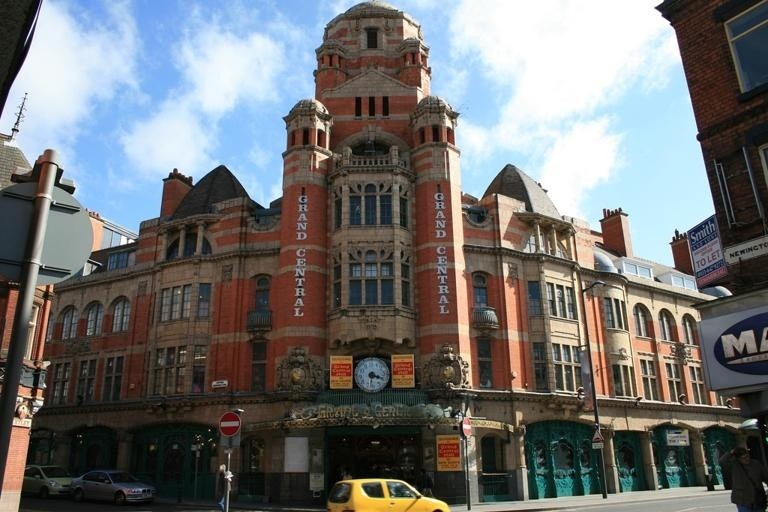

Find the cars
[[324, 477, 449, 512], [67, 469, 157, 507], [17, 464, 74, 499]]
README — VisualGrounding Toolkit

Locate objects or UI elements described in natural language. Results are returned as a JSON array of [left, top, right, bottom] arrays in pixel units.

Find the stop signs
[[460, 417, 473, 437], [215, 412, 242, 438]]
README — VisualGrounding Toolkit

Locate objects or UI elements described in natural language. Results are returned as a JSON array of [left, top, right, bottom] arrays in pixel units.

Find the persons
[[718, 445, 768, 512], [217, 462, 227, 512], [342, 470, 352, 480], [419, 469, 434, 497]]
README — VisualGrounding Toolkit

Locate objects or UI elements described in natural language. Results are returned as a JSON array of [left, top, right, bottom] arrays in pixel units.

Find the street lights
[[566, 258, 610, 500]]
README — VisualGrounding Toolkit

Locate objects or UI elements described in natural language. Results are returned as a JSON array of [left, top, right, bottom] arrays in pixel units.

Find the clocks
[[351, 355, 390, 393]]
